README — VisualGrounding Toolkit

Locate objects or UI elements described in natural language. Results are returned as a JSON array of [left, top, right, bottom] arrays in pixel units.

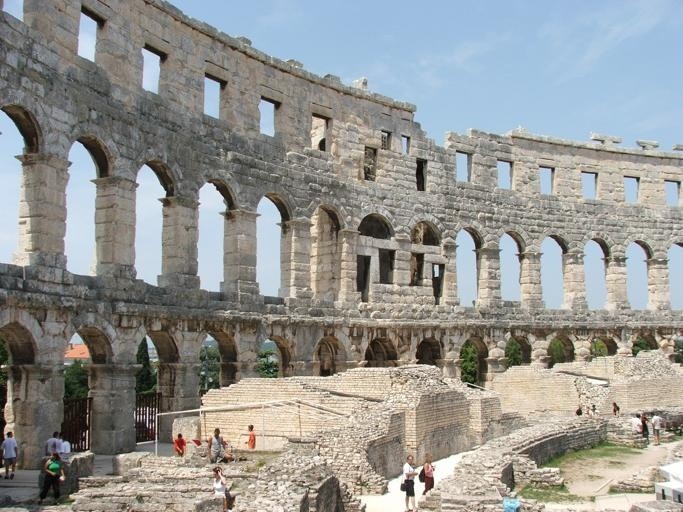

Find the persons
[[575, 405, 583, 415], [207, 428, 232, 464], [53, 432, 64, 455], [174, 433, 186, 458], [632, 413, 642, 433], [45, 431, 59, 456], [501, 490, 520, 511], [612, 402, 619, 416], [0, 431, 19, 480], [209, 466, 228, 512], [591, 403, 596, 415], [402, 454, 417, 512], [641, 414, 651, 445], [244, 424, 256, 450], [422, 452, 435, 496], [650, 412, 663, 445], [37, 452, 66, 506], [59, 434, 71, 454], [584, 407, 590, 416]]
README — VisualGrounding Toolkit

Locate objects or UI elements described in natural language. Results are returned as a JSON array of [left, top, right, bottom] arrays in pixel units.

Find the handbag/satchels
[[400, 484, 406, 491]]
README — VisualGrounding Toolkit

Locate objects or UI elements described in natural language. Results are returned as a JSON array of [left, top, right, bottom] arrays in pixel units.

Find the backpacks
[[419, 467, 425, 482]]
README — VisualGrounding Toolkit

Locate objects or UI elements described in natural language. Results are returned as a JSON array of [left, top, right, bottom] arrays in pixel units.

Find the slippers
[[405, 509, 418, 512]]
